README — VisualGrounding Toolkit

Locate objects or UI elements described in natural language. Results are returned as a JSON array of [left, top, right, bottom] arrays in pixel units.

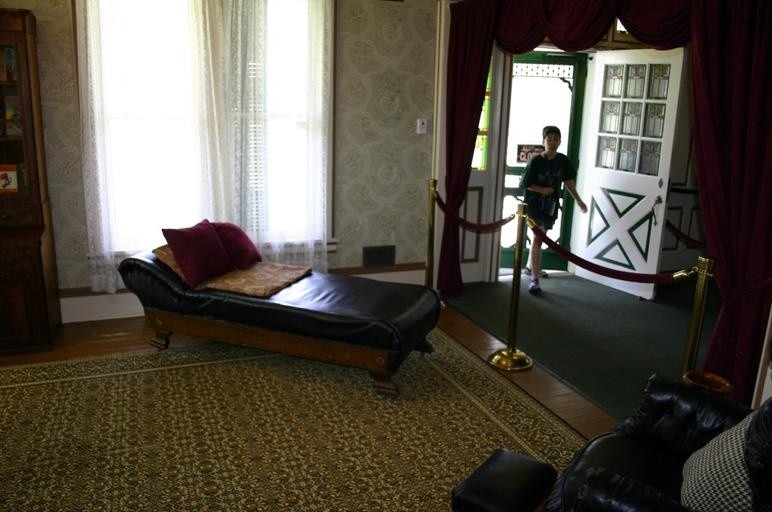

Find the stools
[[450, 448, 558, 512]]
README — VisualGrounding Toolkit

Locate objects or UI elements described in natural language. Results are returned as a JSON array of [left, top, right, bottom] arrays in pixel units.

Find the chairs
[[549, 371, 755, 512], [118, 240, 440, 402]]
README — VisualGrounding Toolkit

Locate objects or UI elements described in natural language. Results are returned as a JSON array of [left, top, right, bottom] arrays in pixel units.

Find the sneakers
[[527, 278, 541, 293], [524, 266, 550, 277]]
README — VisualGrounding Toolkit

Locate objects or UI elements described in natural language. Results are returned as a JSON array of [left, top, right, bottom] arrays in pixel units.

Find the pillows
[[211, 223, 261, 271], [681, 397, 772, 512], [161, 219, 235, 289]]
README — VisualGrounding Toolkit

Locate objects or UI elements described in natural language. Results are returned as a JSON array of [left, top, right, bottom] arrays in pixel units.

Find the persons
[[518, 125, 591, 295]]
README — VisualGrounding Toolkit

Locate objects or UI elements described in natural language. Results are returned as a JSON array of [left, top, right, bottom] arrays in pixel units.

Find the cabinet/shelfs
[[0, 10, 62, 355]]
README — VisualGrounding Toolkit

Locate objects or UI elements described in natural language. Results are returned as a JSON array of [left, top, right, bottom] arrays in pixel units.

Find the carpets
[[0, 327, 589, 512]]
